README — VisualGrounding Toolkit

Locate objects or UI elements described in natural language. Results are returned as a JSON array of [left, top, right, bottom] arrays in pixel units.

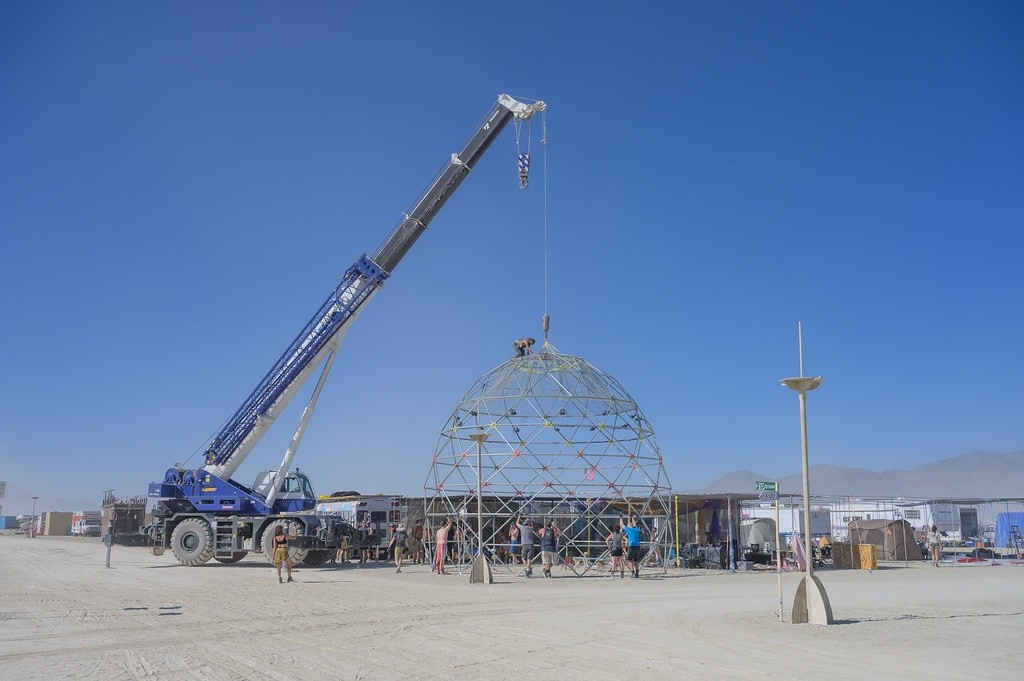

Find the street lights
[[30, 496, 39, 538], [467, 433, 495, 584], [780, 375, 834, 627]]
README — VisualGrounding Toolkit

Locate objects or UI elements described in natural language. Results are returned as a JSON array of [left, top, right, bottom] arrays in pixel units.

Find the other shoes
[[279, 577, 283, 583], [287, 577, 294, 581], [440, 570, 446, 575], [395, 566, 400, 573], [512, 560, 520, 564], [542, 568, 552, 578], [936, 563, 939, 567], [524, 570, 531, 578]]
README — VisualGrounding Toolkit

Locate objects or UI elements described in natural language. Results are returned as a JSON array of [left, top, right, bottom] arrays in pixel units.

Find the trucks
[[71, 510, 103, 537]]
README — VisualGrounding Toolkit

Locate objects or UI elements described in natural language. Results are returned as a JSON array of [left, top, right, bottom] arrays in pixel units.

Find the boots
[[412, 559, 416, 565], [417, 559, 421, 564]]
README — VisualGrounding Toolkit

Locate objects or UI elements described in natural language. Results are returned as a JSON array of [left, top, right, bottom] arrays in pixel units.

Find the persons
[[619, 513, 641, 578], [606, 525, 628, 578], [512, 337, 536, 360], [516, 514, 533, 578], [431, 517, 510, 575], [539, 520, 561, 578], [650, 527, 659, 568], [272, 524, 297, 583], [388, 523, 413, 573], [507, 521, 520, 565]]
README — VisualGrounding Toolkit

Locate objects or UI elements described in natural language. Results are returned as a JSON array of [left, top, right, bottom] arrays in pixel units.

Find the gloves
[[386, 546, 391, 552]]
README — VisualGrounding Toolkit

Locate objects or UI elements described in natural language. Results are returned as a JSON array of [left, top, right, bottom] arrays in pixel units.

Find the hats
[[932, 526, 937, 531], [522, 519, 529, 524], [546, 521, 552, 526], [440, 520, 446, 527], [511, 521, 515, 525], [395, 524, 405, 531], [415, 518, 422, 523], [614, 525, 620, 530]]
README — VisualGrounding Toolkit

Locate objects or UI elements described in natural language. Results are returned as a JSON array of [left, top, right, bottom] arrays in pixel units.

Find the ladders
[[920, 525, 931, 544], [1006, 524, 1024, 554]]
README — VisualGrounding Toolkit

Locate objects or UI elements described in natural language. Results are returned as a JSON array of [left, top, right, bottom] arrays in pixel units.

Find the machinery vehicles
[[97, 89, 549, 568]]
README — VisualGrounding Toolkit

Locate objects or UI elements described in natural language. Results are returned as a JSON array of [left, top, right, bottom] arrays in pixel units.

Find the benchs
[[572, 557, 609, 571]]
